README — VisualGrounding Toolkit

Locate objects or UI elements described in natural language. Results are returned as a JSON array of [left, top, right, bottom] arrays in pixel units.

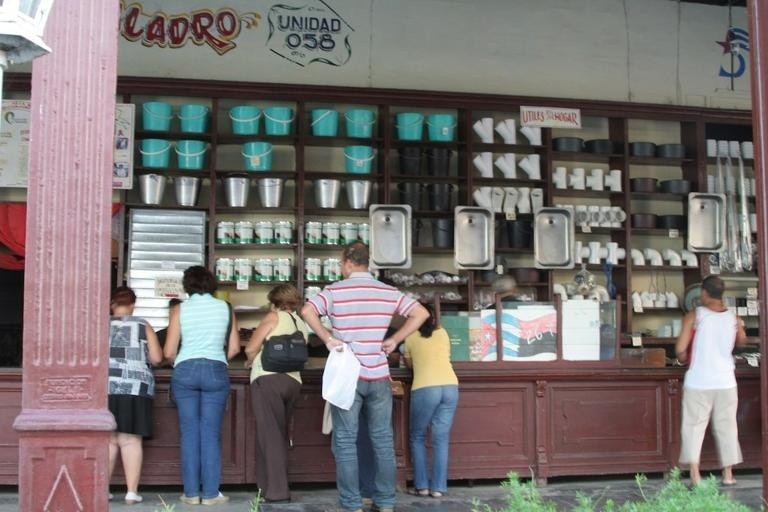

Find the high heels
[[109, 492, 143, 505]]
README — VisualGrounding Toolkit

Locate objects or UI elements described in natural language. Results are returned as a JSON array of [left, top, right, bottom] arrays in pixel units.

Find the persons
[[106, 283, 164, 504], [154, 297, 184, 351], [162, 264, 247, 506], [242, 282, 309, 509], [673, 273, 747, 489], [298, 239, 431, 512], [401, 297, 464, 500]]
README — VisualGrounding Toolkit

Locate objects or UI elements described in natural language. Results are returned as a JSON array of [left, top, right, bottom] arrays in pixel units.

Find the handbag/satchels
[[260, 330, 309, 373]]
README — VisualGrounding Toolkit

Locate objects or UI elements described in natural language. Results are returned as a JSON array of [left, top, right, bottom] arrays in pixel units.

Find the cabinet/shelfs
[[116, 75, 760, 364]]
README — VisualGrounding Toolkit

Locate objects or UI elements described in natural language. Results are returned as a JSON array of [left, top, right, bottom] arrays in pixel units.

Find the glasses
[[337, 259, 352, 265]]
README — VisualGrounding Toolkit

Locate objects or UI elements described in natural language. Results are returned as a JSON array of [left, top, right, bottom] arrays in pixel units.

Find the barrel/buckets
[[494, 219, 531, 249], [137, 102, 377, 210], [396, 111, 457, 249], [215, 220, 380, 282]]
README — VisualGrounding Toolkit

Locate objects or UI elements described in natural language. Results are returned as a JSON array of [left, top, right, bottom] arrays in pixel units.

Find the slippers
[[688, 478, 738, 490]]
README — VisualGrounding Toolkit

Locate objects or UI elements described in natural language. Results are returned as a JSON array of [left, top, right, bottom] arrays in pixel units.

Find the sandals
[[407, 488, 444, 498]]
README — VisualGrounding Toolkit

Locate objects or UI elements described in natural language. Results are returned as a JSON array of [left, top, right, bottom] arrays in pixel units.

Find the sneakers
[[179, 492, 229, 505], [255, 494, 290, 504], [352, 503, 394, 511]]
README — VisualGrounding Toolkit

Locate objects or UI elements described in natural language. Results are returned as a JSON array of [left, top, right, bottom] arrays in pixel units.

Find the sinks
[[453, 205, 496, 271], [533, 206, 576, 270], [686, 191, 727, 253], [368, 203, 413, 271]]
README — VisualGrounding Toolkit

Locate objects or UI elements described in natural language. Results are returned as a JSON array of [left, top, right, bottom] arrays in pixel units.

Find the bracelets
[[323, 335, 336, 344], [675, 358, 690, 367]]
